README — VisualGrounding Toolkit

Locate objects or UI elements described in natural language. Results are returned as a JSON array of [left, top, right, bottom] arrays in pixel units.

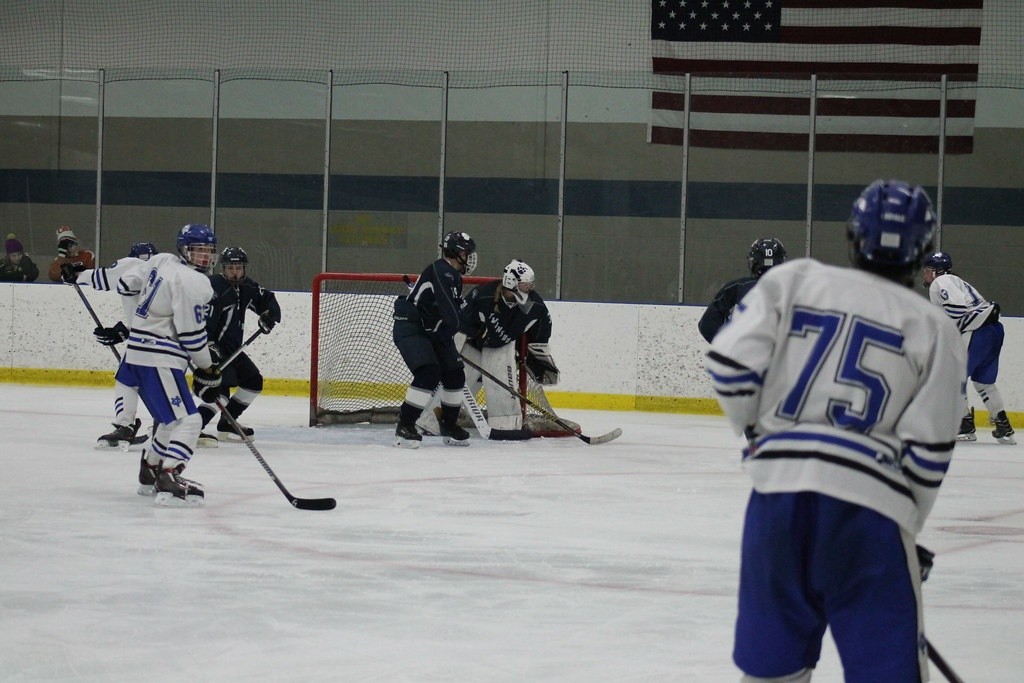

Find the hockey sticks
[[118, 326, 265, 447], [457, 352, 625, 446], [182, 354, 336, 513], [925, 640, 966, 683], [75, 281, 122, 360]]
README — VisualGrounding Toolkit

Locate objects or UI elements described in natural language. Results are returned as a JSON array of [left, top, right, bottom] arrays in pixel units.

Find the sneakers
[[217, 417, 255, 443], [393, 422, 422, 449], [138, 449, 204, 508], [195, 432, 219, 448], [97, 422, 135, 450], [439, 422, 471, 446], [992, 416, 1017, 444], [957, 416, 977, 441]]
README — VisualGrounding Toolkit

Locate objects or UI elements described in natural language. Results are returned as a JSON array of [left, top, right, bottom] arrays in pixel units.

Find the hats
[[56, 226, 78, 245], [6, 233, 23, 254]]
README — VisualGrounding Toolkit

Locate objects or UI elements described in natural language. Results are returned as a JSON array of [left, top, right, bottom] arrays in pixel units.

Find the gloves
[[93, 322, 129, 345], [6, 270, 23, 281], [58, 240, 68, 258], [258, 313, 277, 335], [192, 368, 223, 403], [58, 265, 79, 285]]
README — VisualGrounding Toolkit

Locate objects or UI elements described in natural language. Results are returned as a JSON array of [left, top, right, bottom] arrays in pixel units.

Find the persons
[[191, 247, 281, 447], [923, 251, 1018, 446], [698, 237, 788, 463], [116, 223, 223, 507], [391, 229, 479, 447], [704, 176, 969, 683], [0, 233, 40, 284], [415, 258, 560, 439], [59, 241, 158, 451], [48, 225, 97, 283]]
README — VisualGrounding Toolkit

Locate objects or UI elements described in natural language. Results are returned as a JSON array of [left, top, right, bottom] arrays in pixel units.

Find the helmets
[[923, 249, 952, 278], [177, 224, 219, 273], [748, 238, 787, 279], [848, 180, 939, 277], [444, 232, 477, 275], [502, 259, 534, 305], [220, 247, 248, 281], [129, 242, 158, 259]]
